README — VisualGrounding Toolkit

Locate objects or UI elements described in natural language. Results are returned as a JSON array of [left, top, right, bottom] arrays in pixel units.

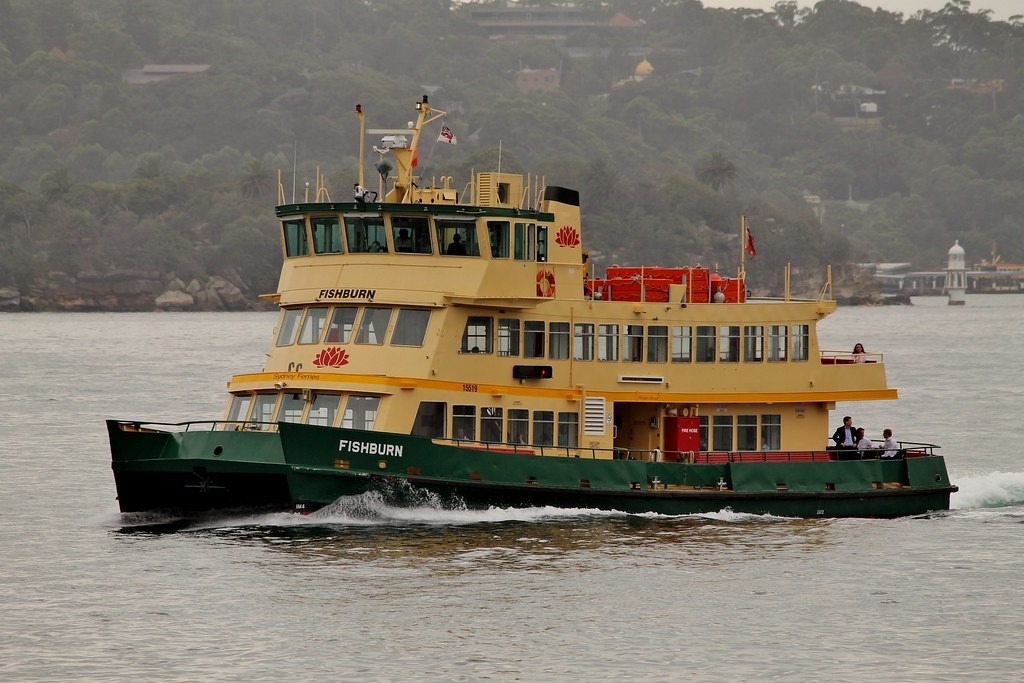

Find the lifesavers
[[537, 268, 555, 296]]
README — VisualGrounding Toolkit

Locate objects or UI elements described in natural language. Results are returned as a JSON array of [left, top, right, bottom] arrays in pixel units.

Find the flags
[[436, 126, 457, 144], [744, 227, 757, 256]]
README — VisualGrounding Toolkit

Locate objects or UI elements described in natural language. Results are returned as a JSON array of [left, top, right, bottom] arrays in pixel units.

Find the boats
[[104, 95, 961, 537]]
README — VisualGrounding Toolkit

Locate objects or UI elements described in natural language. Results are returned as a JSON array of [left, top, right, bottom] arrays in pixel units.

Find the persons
[[833, 416, 861, 460], [851, 343, 866, 363], [856, 428, 873, 460], [762, 438, 770, 450], [366, 228, 469, 257], [455, 427, 470, 440], [879, 429, 901, 460]]
